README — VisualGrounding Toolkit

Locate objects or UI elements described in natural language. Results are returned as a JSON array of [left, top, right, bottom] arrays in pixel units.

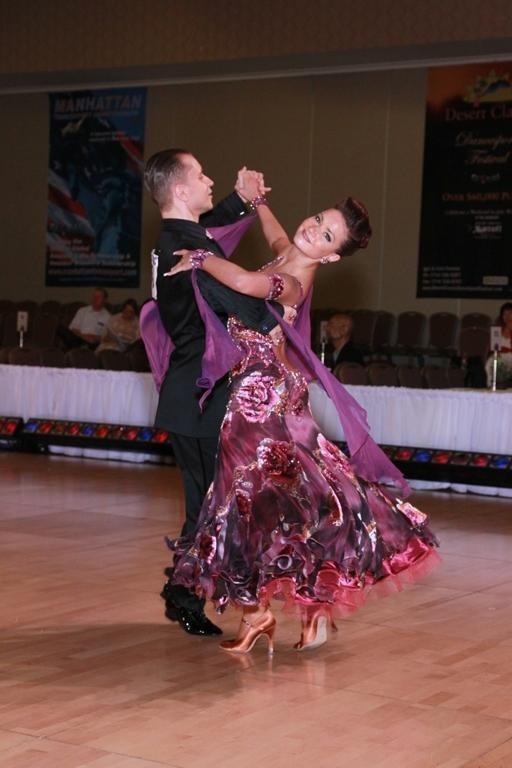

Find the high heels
[[217, 607, 276, 654], [292, 601, 338, 652]]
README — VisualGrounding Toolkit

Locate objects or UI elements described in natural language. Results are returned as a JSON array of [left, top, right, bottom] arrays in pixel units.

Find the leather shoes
[[160, 582, 224, 638]]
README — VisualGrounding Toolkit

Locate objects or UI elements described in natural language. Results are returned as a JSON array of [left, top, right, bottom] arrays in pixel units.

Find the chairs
[[1, 297, 505, 388]]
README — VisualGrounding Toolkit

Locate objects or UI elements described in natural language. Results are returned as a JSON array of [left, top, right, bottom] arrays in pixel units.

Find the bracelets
[[250, 196, 270, 210], [190, 249, 212, 271]]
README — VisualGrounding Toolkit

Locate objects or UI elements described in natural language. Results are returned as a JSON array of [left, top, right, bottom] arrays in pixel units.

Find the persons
[[93, 298, 143, 371], [465, 302, 511, 388], [163, 164, 442, 653], [310, 313, 366, 373], [53, 288, 113, 353], [142, 147, 274, 636]]
